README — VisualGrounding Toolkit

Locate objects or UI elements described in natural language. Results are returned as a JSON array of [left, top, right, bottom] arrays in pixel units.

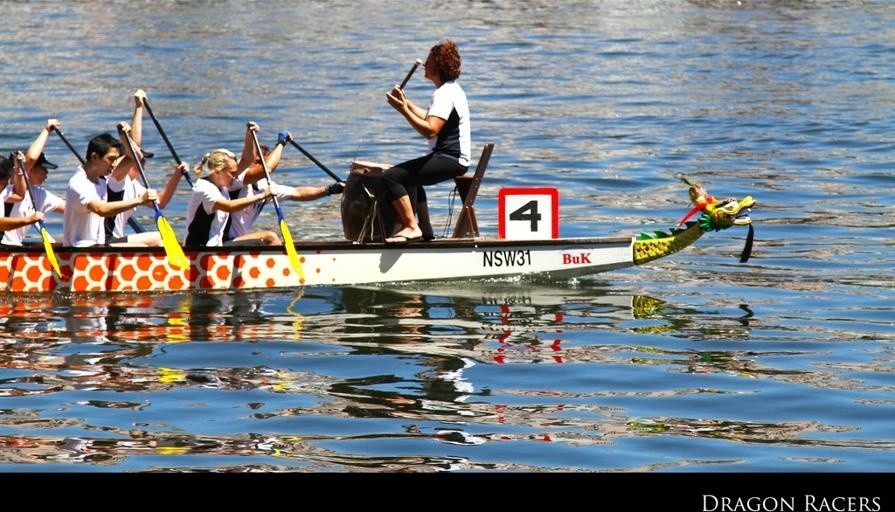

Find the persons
[[373, 40, 472, 244], [0, 148, 47, 246], [0, 119, 68, 243], [182, 121, 283, 246], [60, 120, 157, 247], [223, 128, 348, 240], [104, 89, 189, 246]]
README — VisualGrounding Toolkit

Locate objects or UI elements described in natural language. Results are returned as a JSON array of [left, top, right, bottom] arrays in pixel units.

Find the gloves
[[325, 181, 345, 196], [277, 130, 289, 145]]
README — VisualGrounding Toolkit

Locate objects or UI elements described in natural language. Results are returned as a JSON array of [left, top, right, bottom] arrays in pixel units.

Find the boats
[[1, 169, 756, 297]]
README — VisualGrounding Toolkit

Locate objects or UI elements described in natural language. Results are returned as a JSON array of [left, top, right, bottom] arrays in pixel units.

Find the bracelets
[[15, 172, 24, 177]]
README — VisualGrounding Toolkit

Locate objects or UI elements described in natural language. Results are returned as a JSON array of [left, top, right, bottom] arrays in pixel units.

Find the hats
[[34, 152, 57, 169], [120, 143, 154, 158]]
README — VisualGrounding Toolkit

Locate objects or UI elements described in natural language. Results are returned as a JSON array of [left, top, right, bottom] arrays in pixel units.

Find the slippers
[[383, 234, 424, 246]]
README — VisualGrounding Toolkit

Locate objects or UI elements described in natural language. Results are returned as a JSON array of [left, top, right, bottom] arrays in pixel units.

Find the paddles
[[15, 154, 62, 276], [246, 125, 305, 283], [117, 126, 191, 271]]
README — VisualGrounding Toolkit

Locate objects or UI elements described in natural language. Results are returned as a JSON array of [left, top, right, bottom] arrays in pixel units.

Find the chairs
[[450, 143, 495, 239]]
